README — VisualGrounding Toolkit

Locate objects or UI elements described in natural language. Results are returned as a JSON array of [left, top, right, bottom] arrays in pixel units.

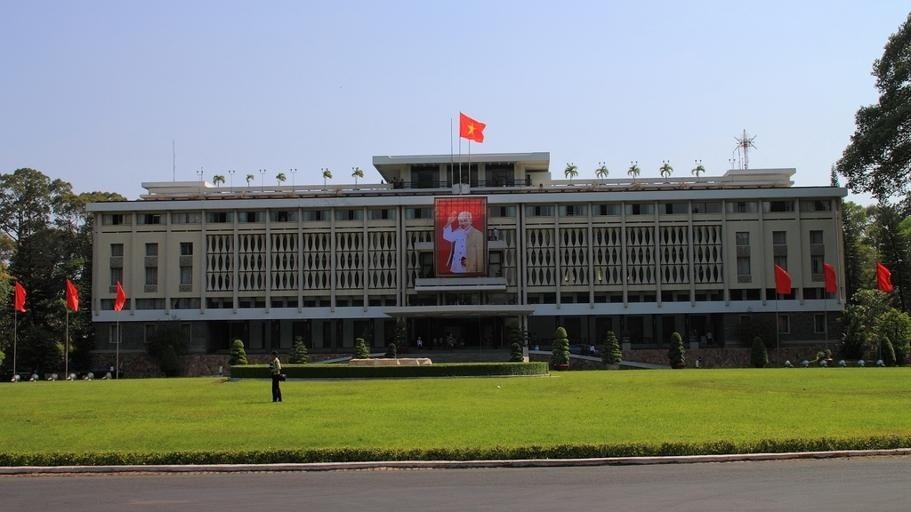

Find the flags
[[112, 281, 126, 311], [15, 281, 27, 313], [877, 262, 894, 292], [776, 264, 793, 295], [66, 279, 79, 313], [824, 263, 837, 293], [459, 112, 487, 143]]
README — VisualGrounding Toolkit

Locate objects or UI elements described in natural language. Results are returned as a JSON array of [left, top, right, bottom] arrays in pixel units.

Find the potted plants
[[817, 350, 833, 365], [602, 330, 622, 370], [668, 332, 687, 369], [549, 327, 570, 370]]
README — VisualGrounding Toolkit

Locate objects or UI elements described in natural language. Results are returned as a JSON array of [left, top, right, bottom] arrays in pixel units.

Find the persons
[[705, 329, 712, 345], [589, 343, 595, 354], [416, 336, 423, 352], [447, 333, 453, 352], [442, 210, 484, 274], [269, 351, 283, 402]]
